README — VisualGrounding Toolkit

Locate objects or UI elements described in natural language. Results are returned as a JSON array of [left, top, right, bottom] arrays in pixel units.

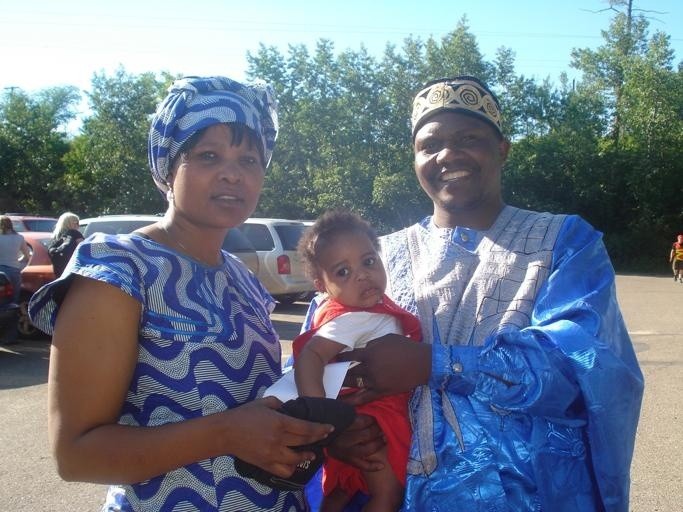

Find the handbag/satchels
[[49, 234, 75, 268], [235, 396, 356, 494]]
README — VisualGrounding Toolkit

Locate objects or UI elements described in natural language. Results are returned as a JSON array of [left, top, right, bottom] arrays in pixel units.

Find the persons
[[0, 216, 30, 346], [40, 211, 85, 277], [297, 214, 421, 512], [286, 77, 644, 512], [669, 234, 683, 283], [48, 74, 334, 512]]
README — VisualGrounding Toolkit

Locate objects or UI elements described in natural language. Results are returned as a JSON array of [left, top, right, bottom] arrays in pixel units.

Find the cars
[[0, 213, 260, 338]]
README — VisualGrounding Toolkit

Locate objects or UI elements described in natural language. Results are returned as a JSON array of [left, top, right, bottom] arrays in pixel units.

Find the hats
[[410, 76, 503, 141]]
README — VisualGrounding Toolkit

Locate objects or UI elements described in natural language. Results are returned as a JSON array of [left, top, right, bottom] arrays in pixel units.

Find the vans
[[242, 218, 318, 307]]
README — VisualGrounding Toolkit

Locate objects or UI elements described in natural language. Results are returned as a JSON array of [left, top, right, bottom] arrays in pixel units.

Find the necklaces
[[157, 218, 224, 272]]
